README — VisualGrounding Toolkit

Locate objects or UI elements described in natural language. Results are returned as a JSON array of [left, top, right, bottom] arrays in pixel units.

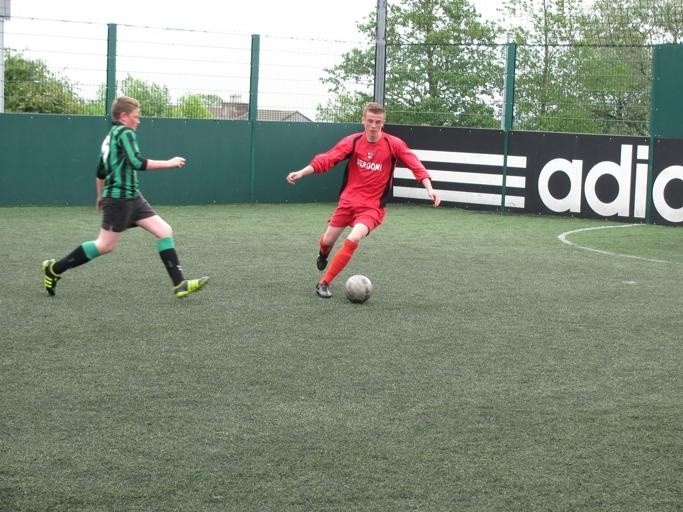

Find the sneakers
[[41, 258, 62, 296], [315, 281, 332, 298], [174, 277, 210, 299], [316, 249, 331, 270]]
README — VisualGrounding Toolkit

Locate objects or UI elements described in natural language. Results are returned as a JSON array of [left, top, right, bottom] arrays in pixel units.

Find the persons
[[40, 96, 211, 298], [287, 103, 441, 298]]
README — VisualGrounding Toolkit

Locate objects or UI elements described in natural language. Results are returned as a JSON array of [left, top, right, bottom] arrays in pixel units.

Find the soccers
[[345, 274, 372, 304]]
